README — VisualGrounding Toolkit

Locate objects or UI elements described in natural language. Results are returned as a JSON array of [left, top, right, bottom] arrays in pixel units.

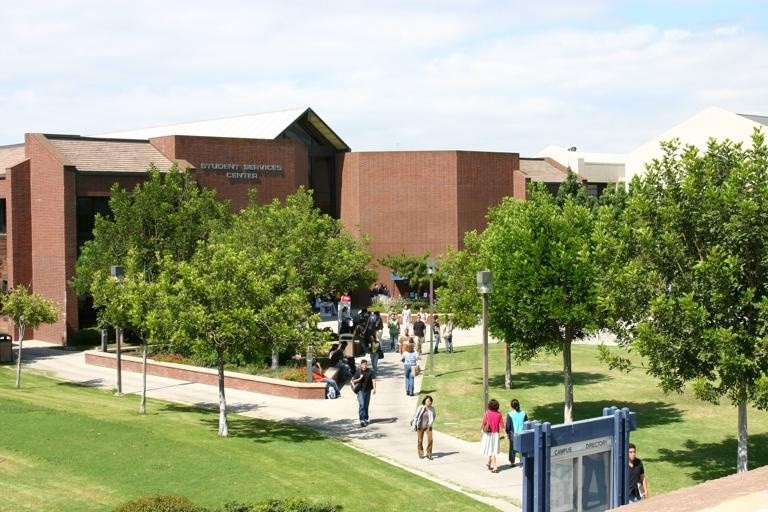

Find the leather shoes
[[406, 390, 413, 394], [487, 464, 497, 473], [361, 419, 368, 425], [419, 454, 433, 461]]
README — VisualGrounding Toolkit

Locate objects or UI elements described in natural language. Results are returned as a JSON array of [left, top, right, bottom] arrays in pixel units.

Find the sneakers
[[508, 462, 515, 467]]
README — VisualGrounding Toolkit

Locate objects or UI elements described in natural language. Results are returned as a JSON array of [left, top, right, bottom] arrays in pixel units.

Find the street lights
[[425, 256, 438, 380], [110, 264, 124, 393], [476, 270, 494, 414]]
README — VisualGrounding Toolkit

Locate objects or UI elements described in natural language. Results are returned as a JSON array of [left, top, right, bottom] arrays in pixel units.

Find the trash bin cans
[[339, 333, 355, 358], [0, 333, 12, 363]]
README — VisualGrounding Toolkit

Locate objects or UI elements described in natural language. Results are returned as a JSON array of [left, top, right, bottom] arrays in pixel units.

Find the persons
[[582, 451, 607, 508], [315, 293, 321, 310], [482, 398, 529, 473], [311, 304, 454, 427], [412, 395, 437, 460], [629, 443, 648, 504], [370, 282, 388, 297]]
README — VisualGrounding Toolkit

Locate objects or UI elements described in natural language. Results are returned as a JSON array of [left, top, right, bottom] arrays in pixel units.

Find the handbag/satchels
[[411, 365, 420, 378], [483, 424, 491, 433], [378, 348, 384, 358], [409, 419, 420, 432], [349, 378, 361, 392]]
